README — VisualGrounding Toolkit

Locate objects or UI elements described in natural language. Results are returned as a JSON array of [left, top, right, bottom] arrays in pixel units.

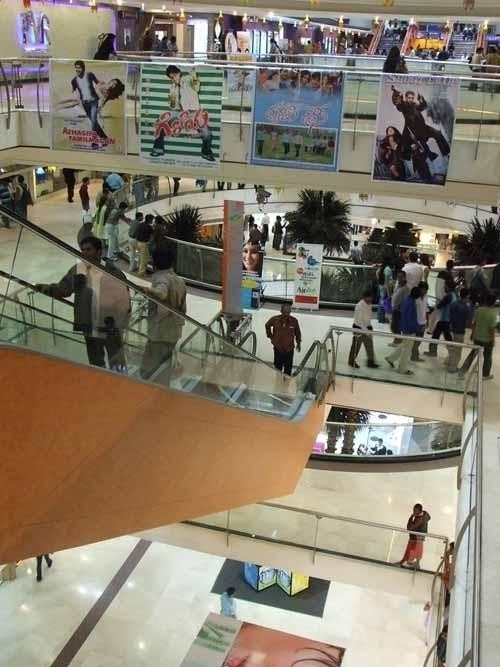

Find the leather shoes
[[348, 361, 359, 369], [384, 357, 395, 368], [398, 370, 414, 374], [424, 351, 437, 357]]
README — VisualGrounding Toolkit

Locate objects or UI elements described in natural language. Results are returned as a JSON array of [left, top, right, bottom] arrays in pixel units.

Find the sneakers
[[150, 151, 164, 157], [201, 153, 216, 162]]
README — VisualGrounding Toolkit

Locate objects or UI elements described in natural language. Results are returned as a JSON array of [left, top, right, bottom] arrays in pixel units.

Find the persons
[[143, 31, 178, 56], [348, 241, 362, 262], [63, 168, 76, 202], [79, 173, 165, 277], [0, 176, 30, 229], [454, 24, 477, 42], [150, 65, 216, 161], [270, 39, 279, 62], [217, 181, 245, 191], [35, 236, 130, 375], [409, 44, 455, 61], [383, 47, 408, 74], [36, 550, 53, 581], [443, 541, 454, 609], [374, 49, 387, 56], [400, 504, 430, 568], [435, 625, 448, 665], [468, 46, 500, 92], [140, 241, 187, 388], [96, 33, 116, 60], [55, 60, 125, 150], [346, 248, 500, 383], [255, 68, 343, 158], [241, 241, 262, 272], [247, 213, 286, 251], [384, 20, 408, 42], [201, 180, 208, 193], [379, 84, 451, 181], [220, 587, 237, 618], [304, 40, 321, 54], [370, 439, 387, 456], [265, 304, 302, 376], [173, 177, 181, 196]]
